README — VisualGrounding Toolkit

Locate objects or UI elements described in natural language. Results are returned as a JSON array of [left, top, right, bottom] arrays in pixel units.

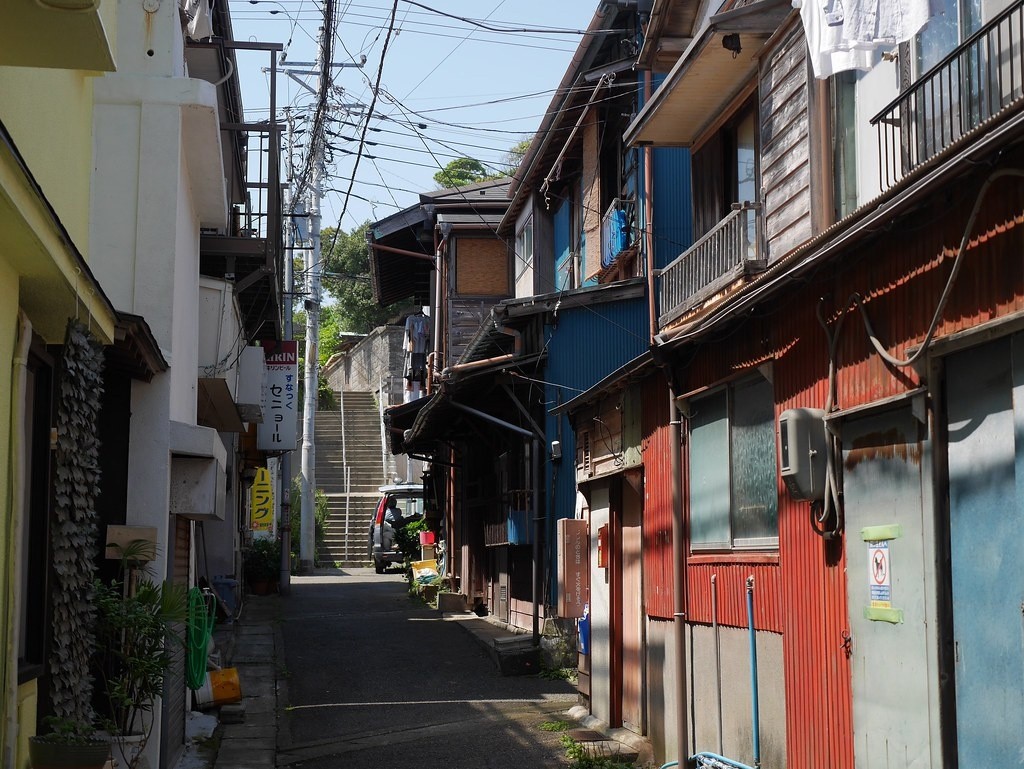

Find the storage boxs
[[422, 545, 435, 560]]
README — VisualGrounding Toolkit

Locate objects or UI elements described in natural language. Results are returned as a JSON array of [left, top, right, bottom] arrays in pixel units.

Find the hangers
[[414, 306, 427, 317]]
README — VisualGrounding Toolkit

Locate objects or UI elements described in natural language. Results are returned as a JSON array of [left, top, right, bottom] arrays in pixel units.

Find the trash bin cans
[[410, 559, 437, 582], [212, 578, 238, 622]]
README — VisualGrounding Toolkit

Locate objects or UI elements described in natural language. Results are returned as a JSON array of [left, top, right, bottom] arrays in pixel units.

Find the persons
[[384, 498, 402, 521]]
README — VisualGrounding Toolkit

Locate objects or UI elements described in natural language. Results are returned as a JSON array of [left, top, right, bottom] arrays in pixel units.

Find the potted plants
[[93, 536, 211, 769], [29, 716, 113, 769], [244, 536, 282, 596]]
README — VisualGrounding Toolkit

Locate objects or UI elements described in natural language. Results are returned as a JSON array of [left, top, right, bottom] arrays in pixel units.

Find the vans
[[368, 484, 426, 575]]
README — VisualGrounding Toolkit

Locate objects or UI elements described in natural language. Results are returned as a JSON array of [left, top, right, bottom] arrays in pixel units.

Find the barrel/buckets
[[194, 668, 241, 710]]
